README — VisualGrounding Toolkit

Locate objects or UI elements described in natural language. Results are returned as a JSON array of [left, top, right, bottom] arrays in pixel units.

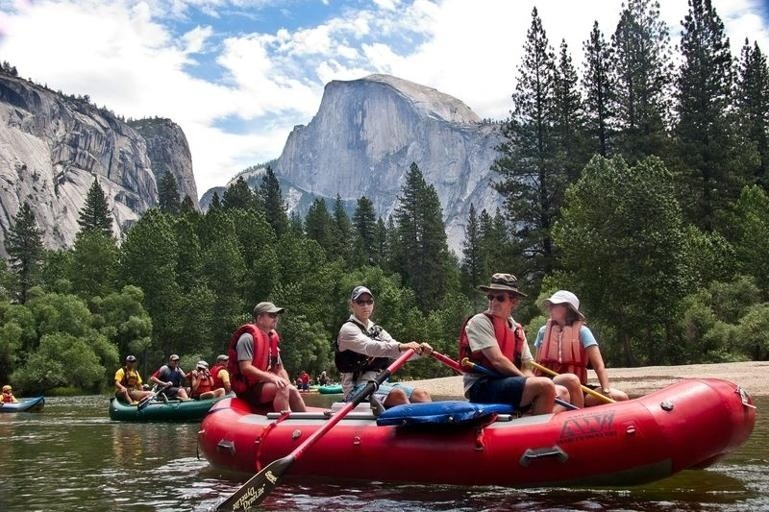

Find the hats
[[479, 273, 528, 298], [253, 301, 285, 318], [168, 354, 180, 361], [125, 355, 135, 362], [216, 354, 229, 363], [351, 286, 375, 303], [195, 361, 209, 370], [542, 290, 585, 321]]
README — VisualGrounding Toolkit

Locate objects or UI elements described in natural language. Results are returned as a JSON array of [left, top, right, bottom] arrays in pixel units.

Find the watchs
[[602, 385, 611, 395]]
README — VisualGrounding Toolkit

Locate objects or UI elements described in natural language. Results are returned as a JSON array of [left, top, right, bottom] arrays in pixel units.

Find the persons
[[533, 290, 630, 409], [150, 354, 192, 402], [334, 286, 433, 407], [188, 360, 226, 400], [227, 302, 307, 411], [210, 354, 232, 395], [0, 385, 18, 405], [459, 273, 571, 415], [114, 355, 151, 406], [293, 369, 331, 394]]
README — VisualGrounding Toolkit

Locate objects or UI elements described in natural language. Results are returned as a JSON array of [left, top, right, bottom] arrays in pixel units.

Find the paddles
[[137, 383, 172, 411], [216, 346, 418, 512]]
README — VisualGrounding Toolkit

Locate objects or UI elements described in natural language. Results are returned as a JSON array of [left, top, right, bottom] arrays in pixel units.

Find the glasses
[[484, 293, 511, 304], [352, 299, 375, 306], [264, 313, 278, 319], [128, 360, 135, 363]]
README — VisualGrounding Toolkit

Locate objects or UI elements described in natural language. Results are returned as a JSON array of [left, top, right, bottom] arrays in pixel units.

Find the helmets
[[1, 385, 12, 393]]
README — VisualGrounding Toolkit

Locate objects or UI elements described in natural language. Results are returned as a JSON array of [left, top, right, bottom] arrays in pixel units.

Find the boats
[[200, 377, 758, 486], [0, 395, 46, 413], [110, 391, 237, 423], [293, 384, 344, 394]]
